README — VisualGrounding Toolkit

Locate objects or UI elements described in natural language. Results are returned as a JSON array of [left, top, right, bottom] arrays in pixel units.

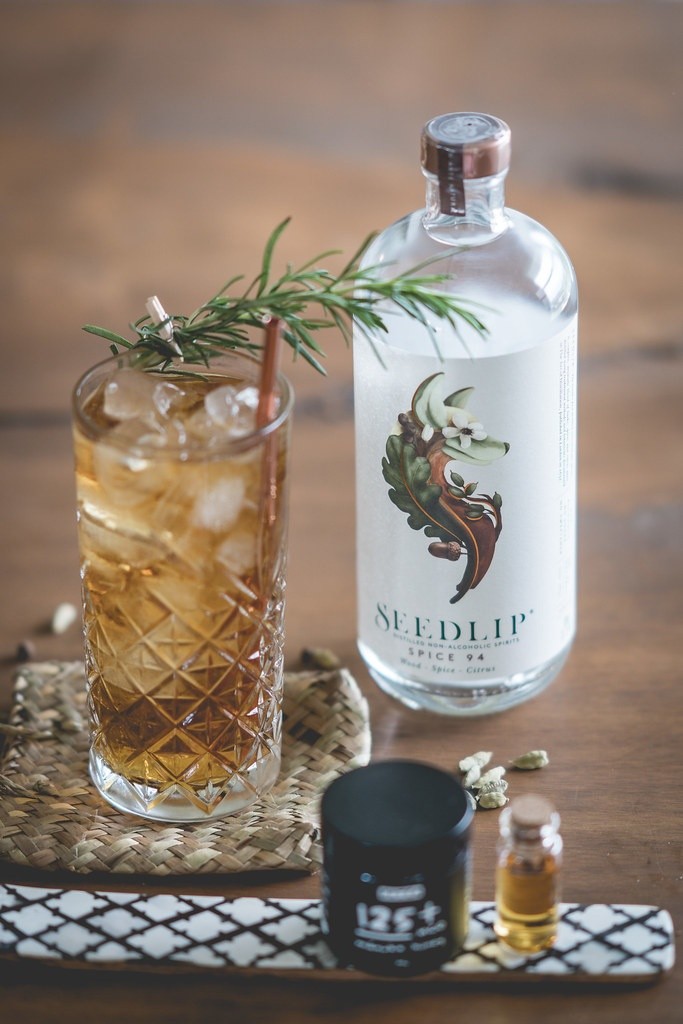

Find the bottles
[[493, 793, 561, 955], [353, 112, 576, 712]]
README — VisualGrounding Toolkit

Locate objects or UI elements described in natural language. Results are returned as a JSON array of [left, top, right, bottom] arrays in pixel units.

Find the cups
[[71, 345, 293, 824]]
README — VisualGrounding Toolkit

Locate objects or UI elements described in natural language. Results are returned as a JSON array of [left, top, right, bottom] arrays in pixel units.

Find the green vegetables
[[78, 214, 493, 385]]
